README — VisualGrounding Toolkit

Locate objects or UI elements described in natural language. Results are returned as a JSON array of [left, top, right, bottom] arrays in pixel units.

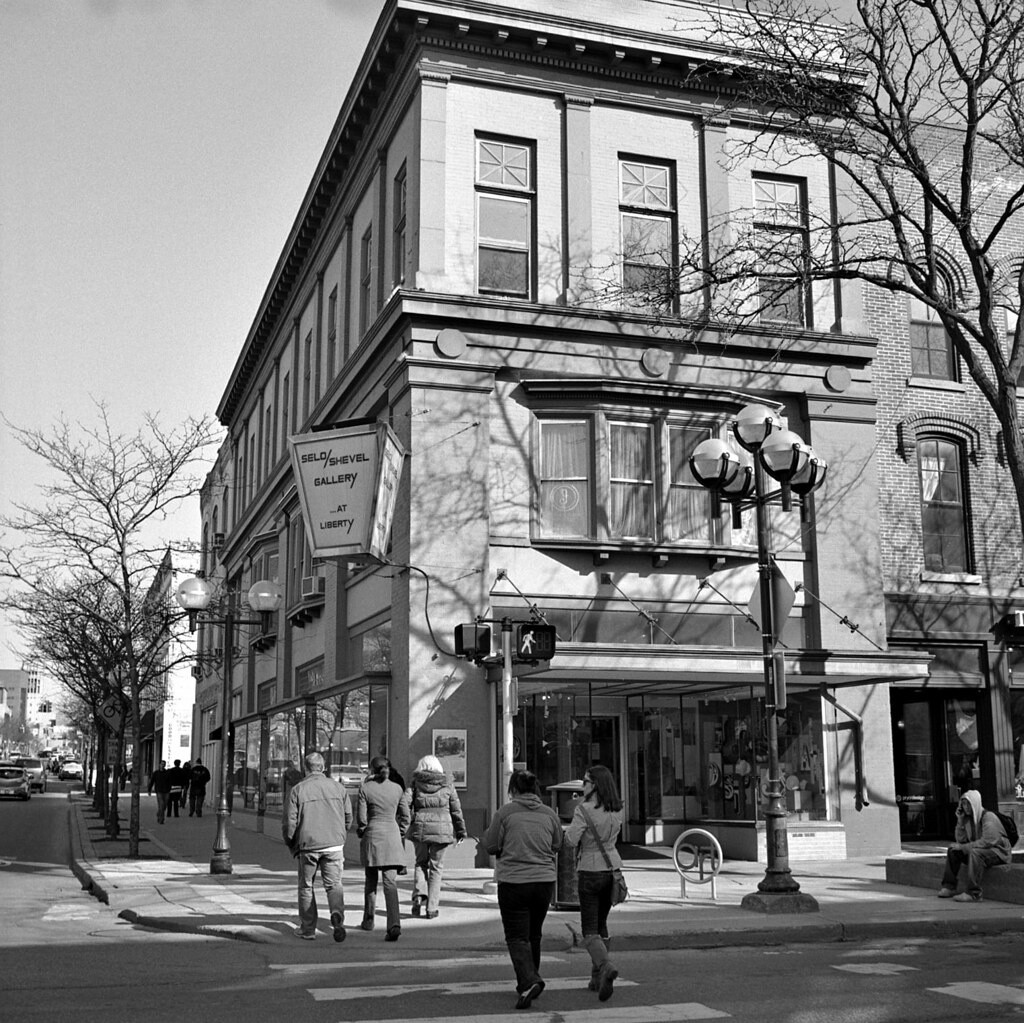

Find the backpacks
[[961, 808, 1019, 847]]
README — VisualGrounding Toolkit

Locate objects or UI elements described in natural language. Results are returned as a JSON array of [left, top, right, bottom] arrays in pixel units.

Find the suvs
[[17, 758, 48, 793]]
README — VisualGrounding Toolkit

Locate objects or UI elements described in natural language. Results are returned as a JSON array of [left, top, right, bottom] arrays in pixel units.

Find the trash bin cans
[[546, 779, 584, 911]]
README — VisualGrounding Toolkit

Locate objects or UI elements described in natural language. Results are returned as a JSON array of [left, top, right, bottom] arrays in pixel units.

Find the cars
[[36, 747, 84, 781], [230, 748, 296, 791], [0, 750, 22, 767], [331, 764, 369, 787], [0, 767, 32, 801]]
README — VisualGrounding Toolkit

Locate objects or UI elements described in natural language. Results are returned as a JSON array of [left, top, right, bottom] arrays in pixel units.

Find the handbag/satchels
[[611, 871, 628, 905]]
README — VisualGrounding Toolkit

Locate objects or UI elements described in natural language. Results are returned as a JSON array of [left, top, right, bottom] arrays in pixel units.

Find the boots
[[582, 936, 612, 990], [583, 935, 619, 1001]]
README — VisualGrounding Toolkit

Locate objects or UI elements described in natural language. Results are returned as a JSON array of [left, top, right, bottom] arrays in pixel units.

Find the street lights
[[688, 404, 828, 914], [175, 577, 281, 876]]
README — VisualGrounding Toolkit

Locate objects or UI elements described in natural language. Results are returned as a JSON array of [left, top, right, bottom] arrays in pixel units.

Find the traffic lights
[[454, 622, 491, 659], [46, 701, 53, 713], [516, 622, 557, 660]]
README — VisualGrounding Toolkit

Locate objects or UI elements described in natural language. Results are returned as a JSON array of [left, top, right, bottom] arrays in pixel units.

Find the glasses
[[582, 778, 595, 786]]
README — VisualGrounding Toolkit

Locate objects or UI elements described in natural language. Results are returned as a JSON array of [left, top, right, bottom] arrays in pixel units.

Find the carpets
[[616, 842, 673, 860]]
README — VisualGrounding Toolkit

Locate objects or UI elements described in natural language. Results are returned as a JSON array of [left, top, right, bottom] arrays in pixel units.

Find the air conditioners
[[301, 575, 325, 597], [210, 532, 224, 551], [192, 666, 202, 677], [1014, 611, 1024, 627], [214, 648, 223, 660]]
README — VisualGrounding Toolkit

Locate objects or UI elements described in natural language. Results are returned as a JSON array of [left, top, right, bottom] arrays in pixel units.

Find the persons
[[280, 753, 353, 942], [565, 765, 624, 1002], [938, 790, 1011, 902], [235, 758, 260, 809], [120, 765, 129, 790], [147, 758, 209, 824], [487, 770, 563, 1010], [357, 755, 466, 939]]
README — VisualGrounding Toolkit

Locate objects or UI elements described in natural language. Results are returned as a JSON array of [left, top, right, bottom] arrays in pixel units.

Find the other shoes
[[426, 909, 439, 919], [953, 892, 983, 903], [361, 920, 374, 930], [385, 927, 400, 942], [533, 982, 545, 1000], [937, 887, 964, 898], [516, 983, 540, 1010], [412, 896, 422, 917], [331, 910, 346, 942], [293, 928, 315, 940]]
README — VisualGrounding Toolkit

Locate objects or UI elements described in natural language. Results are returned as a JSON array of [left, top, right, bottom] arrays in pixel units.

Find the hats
[[196, 758, 202, 764]]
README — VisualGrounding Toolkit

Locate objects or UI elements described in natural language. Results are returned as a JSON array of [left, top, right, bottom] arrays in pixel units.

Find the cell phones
[[958, 806, 964, 815]]
